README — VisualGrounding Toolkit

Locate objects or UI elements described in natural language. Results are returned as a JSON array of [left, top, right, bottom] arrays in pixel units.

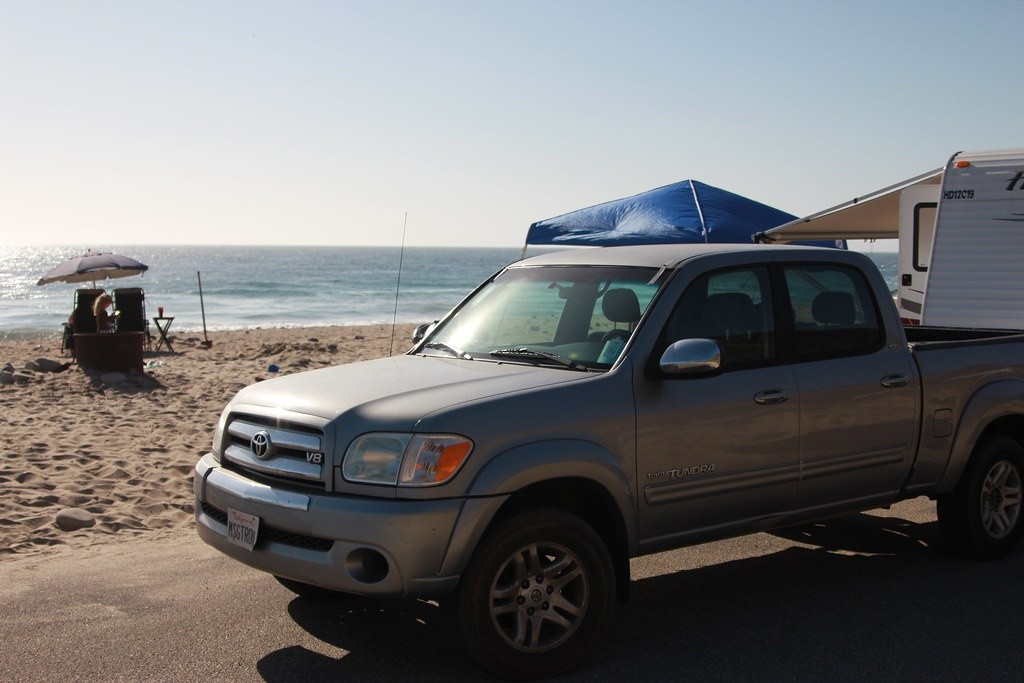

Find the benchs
[[753, 291, 877, 356]]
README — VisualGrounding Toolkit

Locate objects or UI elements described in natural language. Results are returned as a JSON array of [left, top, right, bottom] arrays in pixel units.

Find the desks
[[154, 317, 175, 354]]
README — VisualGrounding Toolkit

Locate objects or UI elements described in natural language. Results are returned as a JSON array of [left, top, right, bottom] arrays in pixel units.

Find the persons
[[92, 292, 120, 333]]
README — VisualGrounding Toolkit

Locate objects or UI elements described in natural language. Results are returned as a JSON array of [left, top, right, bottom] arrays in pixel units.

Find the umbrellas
[[35, 247, 149, 289]]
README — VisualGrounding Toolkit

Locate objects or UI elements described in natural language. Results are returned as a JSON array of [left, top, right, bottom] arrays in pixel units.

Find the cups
[[158, 307, 163, 318]]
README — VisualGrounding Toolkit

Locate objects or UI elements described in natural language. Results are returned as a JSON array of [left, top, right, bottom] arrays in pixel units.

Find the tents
[[519, 180, 849, 262]]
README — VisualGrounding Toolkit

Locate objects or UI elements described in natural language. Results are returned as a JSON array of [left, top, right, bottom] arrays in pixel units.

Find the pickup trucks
[[193, 241, 1024, 683]]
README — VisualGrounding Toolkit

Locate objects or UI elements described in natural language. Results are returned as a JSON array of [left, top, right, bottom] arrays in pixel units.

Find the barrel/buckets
[[269, 364, 279, 373]]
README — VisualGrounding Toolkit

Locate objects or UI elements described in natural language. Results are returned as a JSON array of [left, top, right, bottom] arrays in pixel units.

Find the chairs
[[700, 292, 764, 371], [111, 287, 151, 354], [60, 288, 107, 364], [587, 288, 641, 342]]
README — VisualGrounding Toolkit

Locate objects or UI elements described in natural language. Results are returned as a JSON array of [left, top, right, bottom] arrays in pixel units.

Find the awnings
[[751, 166, 947, 245]]
